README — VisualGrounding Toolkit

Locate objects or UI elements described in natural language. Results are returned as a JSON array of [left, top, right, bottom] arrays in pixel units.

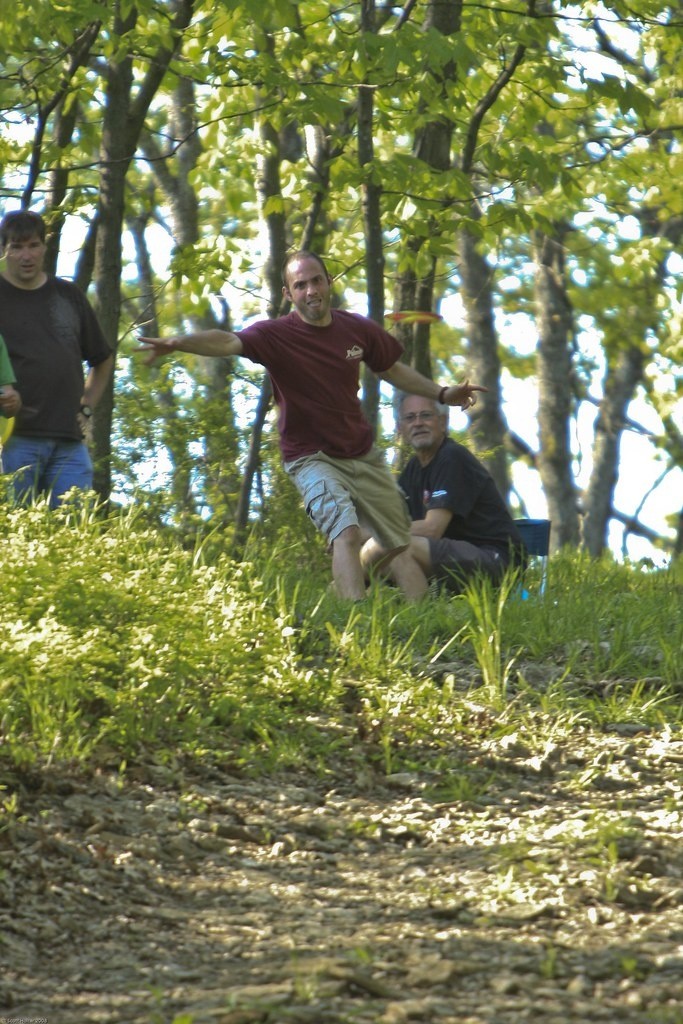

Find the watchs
[[79, 404, 92, 418]]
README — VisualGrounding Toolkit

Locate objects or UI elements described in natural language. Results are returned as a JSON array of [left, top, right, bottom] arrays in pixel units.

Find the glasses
[[402, 411, 434, 423]]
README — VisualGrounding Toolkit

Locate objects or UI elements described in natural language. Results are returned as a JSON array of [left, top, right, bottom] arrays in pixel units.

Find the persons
[[327, 391, 528, 591], [0, 211, 113, 525], [134, 251, 487, 602]]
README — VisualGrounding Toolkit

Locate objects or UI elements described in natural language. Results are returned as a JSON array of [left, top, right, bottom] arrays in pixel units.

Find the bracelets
[[438, 387, 456, 405]]
